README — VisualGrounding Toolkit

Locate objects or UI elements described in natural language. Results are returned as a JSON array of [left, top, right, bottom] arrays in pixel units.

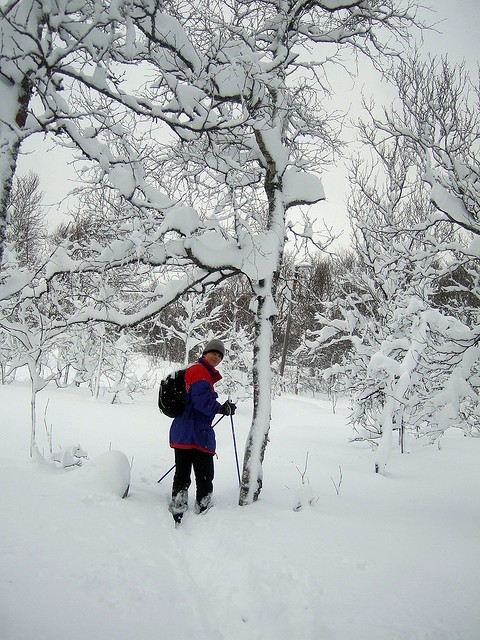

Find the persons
[[169, 338, 236, 523]]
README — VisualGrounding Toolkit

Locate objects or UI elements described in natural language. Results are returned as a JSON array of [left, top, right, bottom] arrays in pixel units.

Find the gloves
[[222, 400, 237, 416]]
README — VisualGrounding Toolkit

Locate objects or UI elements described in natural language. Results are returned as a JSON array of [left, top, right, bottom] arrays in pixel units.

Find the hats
[[203, 339, 225, 358]]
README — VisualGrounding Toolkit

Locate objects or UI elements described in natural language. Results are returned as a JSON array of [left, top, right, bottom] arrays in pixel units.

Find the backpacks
[[158, 369, 186, 418]]
